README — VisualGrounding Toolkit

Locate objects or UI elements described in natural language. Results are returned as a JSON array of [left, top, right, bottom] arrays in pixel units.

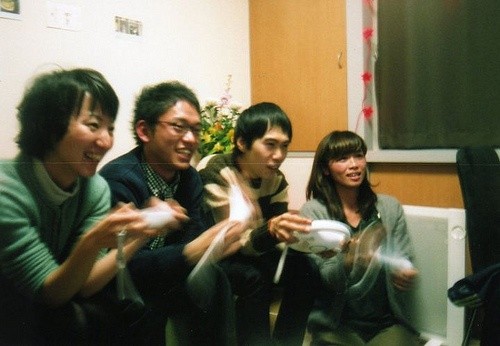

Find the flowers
[[194, 99, 245, 158]]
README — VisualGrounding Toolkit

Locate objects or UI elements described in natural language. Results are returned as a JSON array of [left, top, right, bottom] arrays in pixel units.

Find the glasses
[[155, 121, 203, 136]]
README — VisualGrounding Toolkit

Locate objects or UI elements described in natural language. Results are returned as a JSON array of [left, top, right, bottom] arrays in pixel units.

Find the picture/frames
[[0, 0, 23, 21]]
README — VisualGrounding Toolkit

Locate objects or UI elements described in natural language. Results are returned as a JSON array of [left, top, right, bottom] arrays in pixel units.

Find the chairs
[[456, 145, 498, 344]]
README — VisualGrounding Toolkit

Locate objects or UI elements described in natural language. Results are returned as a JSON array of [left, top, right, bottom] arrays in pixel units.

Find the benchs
[[401, 204, 468, 346]]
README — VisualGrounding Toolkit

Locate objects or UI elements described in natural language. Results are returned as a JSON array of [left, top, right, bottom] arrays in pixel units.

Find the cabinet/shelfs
[[250, 0, 350, 153]]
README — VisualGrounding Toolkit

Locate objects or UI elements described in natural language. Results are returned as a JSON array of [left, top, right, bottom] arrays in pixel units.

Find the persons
[[0, 68, 192, 346], [95, 82, 250, 346], [196, 102, 311, 345], [297, 130, 418, 345]]
